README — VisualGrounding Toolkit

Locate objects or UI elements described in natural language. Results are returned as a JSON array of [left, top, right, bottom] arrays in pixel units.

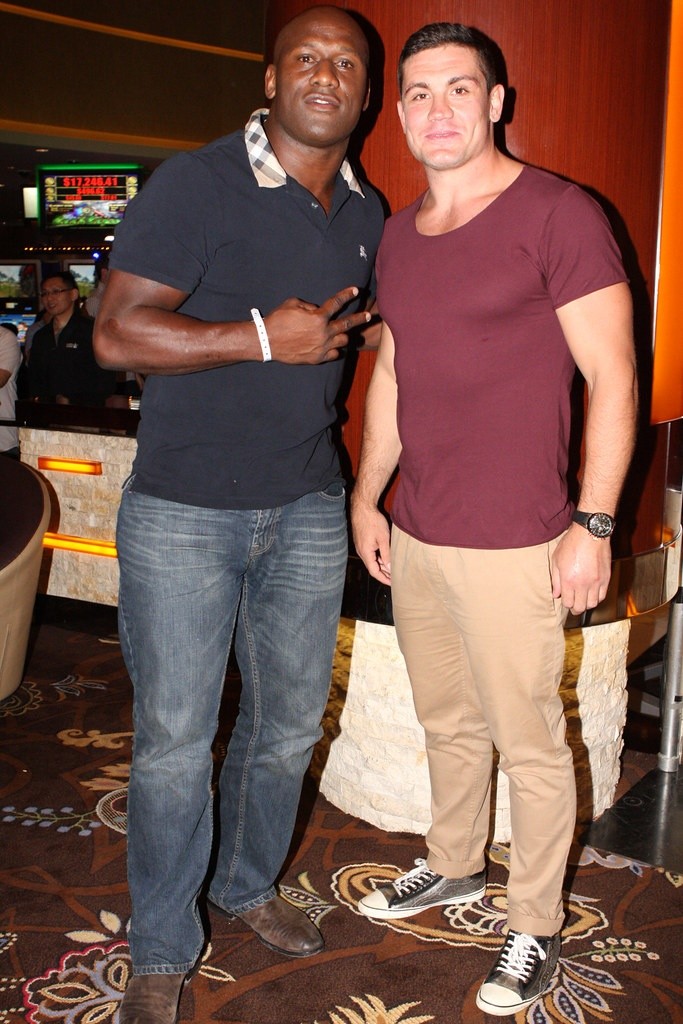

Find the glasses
[[36, 288, 71, 299]]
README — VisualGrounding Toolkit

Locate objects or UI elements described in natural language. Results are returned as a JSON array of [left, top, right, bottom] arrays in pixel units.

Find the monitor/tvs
[[0, 259, 43, 314], [37, 163, 143, 234], [63, 259, 98, 309]]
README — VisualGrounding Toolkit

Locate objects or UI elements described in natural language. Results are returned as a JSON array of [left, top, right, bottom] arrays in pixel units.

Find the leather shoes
[[119, 959, 190, 1024], [208, 882, 324, 960]]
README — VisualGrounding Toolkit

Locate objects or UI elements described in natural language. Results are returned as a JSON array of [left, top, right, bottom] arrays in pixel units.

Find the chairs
[[1, 456, 51, 702]]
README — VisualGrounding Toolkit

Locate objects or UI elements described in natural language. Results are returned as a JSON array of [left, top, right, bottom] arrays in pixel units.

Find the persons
[[352, 22, 638, 1016], [93, 9, 392, 1024], [0, 251, 109, 461]]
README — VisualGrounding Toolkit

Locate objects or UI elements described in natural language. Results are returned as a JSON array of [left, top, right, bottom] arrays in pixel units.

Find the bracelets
[[251, 308, 272, 362]]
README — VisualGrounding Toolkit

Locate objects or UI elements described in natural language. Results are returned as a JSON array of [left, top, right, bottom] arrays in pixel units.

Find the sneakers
[[358, 860, 485, 921], [475, 928, 560, 1018]]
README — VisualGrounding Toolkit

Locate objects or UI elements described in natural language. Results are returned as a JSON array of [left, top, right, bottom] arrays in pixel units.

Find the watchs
[[573, 510, 616, 539]]
